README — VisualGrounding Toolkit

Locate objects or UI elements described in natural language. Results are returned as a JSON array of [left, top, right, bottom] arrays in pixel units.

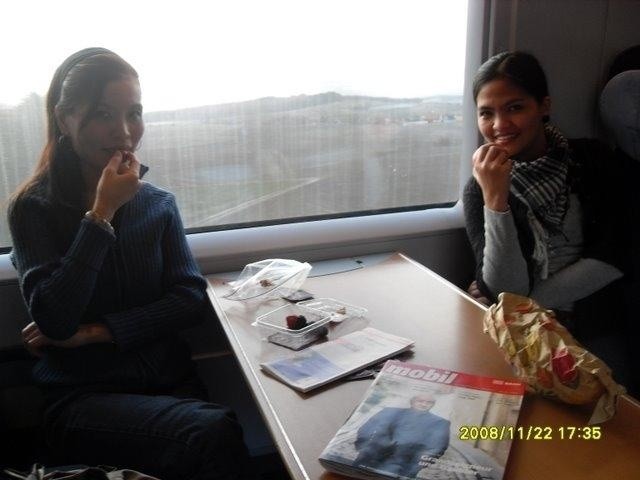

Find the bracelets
[[84, 208, 115, 233]]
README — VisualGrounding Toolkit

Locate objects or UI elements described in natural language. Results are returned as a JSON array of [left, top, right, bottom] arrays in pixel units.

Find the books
[[259, 326, 417, 397], [319, 360, 527, 479]]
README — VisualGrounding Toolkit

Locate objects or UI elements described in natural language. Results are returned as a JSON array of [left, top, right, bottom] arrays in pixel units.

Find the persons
[[4, 47, 259, 479], [459, 49, 638, 401], [352, 393, 453, 479]]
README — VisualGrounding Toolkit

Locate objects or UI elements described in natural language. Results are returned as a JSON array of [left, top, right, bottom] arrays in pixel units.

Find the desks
[[201, 250, 640, 480]]
